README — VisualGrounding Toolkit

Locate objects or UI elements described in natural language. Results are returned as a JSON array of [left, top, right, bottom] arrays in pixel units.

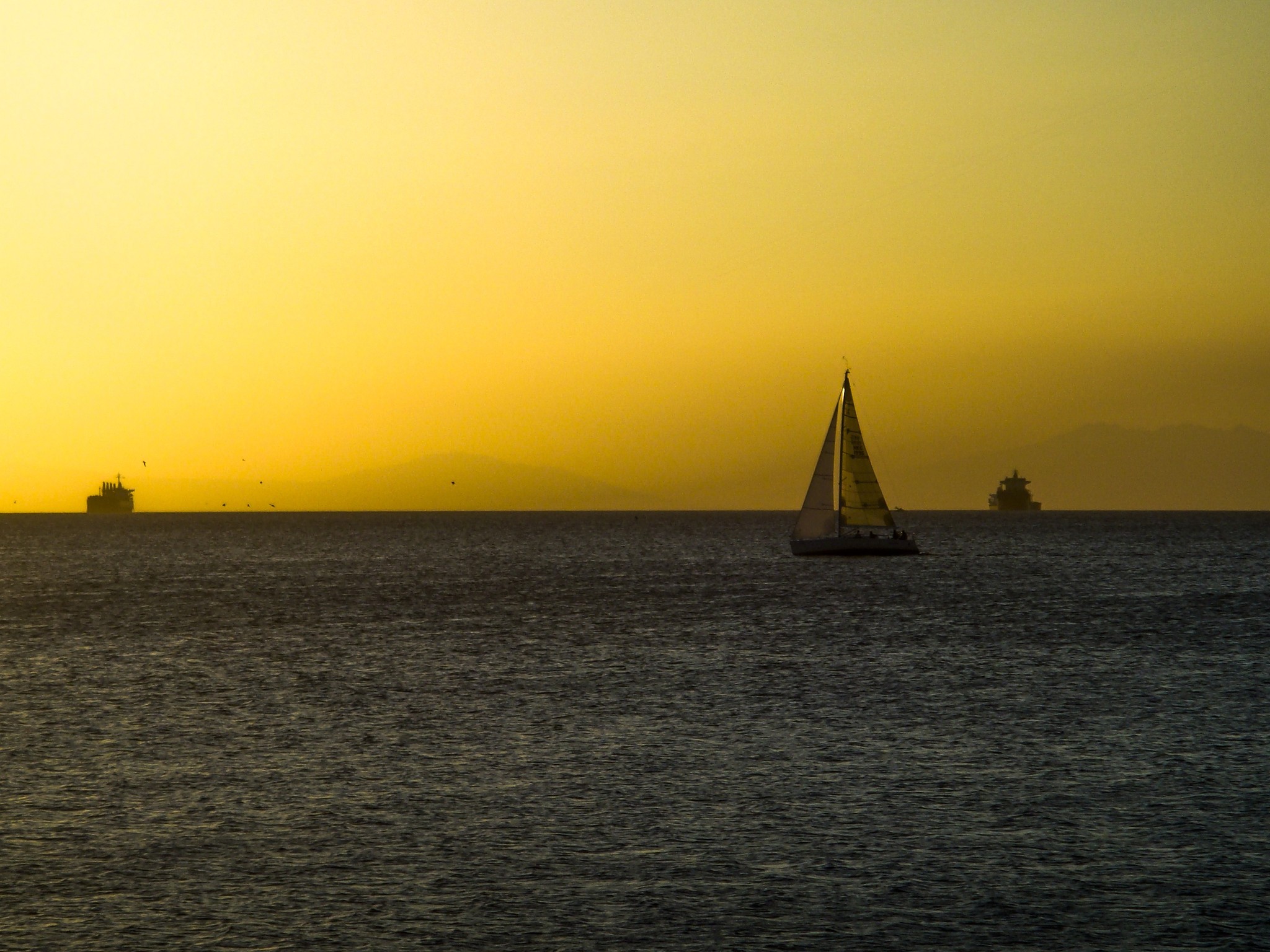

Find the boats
[[988, 468, 1045, 510], [86, 474, 135, 514]]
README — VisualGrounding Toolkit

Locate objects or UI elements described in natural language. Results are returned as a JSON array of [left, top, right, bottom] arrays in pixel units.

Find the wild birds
[[242, 459, 245, 461], [269, 503, 275, 507], [221, 503, 226, 507], [142, 461, 146, 468], [247, 504, 250, 507], [451, 482, 455, 485], [260, 481, 262, 484], [894, 506, 904, 510]]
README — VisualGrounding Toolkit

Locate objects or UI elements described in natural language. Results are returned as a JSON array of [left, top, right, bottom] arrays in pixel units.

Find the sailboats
[[787, 364, 922, 554]]
